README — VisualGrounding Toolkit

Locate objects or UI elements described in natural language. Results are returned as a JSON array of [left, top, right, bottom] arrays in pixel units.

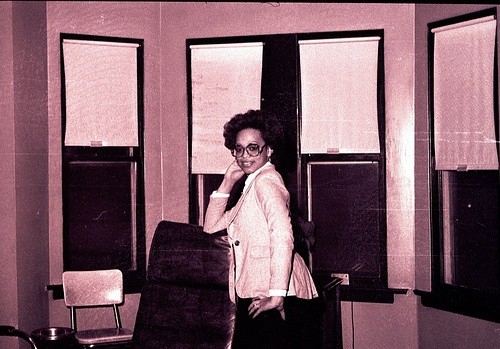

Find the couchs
[[130, 221, 237, 349]]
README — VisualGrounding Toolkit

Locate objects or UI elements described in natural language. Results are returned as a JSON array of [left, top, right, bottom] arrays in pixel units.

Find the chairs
[[62, 269, 133, 349]]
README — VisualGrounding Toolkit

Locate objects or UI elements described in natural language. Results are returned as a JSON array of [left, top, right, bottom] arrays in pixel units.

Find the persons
[[201, 108, 319, 349]]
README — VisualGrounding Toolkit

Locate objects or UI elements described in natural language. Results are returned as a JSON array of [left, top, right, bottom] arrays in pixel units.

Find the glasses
[[231, 142, 268, 158]]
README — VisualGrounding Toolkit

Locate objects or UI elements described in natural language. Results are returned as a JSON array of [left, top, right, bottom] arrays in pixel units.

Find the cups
[[31, 327, 74, 349]]
